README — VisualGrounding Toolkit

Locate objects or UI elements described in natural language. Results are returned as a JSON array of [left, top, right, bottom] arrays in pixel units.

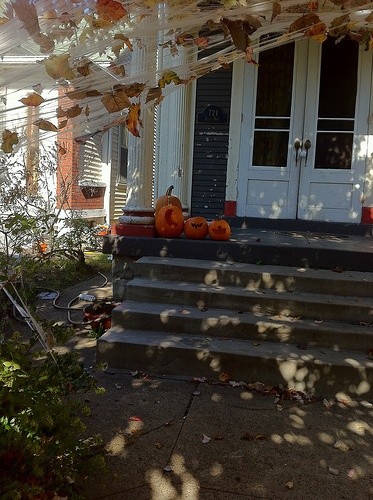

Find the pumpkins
[[154, 185, 182, 214]]
